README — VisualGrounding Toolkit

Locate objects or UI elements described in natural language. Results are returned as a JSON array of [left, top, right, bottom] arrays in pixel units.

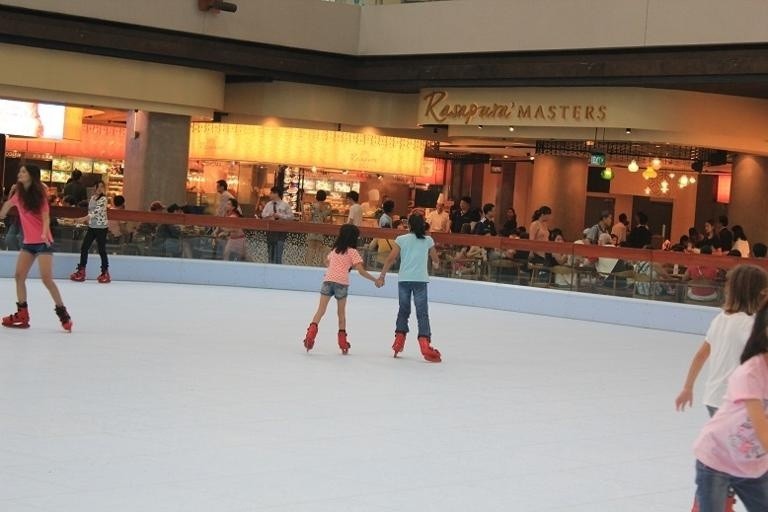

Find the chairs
[[0, 217, 765, 319]]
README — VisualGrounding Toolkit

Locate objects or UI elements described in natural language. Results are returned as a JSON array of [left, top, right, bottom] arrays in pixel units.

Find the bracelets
[[380, 272, 386, 277]]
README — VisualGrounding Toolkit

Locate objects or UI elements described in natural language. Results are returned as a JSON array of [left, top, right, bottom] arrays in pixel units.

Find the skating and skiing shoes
[[56, 304, 72, 332], [70, 264, 85, 281], [97, 271, 111, 283], [2, 303, 29, 326], [338, 330, 350, 352], [392, 332, 406, 358], [418, 337, 441, 362], [304, 322, 318, 351]]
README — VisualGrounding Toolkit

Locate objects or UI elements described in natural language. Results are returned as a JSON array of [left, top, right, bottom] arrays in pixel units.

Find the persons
[[1, 168, 291, 264], [374, 212, 442, 365], [426, 193, 531, 284], [675, 265, 766, 512], [694, 303, 766, 512], [528, 204, 668, 298], [308, 189, 407, 272], [71, 180, 112, 284], [1, 165, 74, 333], [671, 215, 767, 301], [301, 224, 377, 355]]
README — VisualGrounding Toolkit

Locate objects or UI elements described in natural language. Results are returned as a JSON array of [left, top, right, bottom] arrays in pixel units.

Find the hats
[[151, 201, 166, 209], [437, 193, 444, 204]]
